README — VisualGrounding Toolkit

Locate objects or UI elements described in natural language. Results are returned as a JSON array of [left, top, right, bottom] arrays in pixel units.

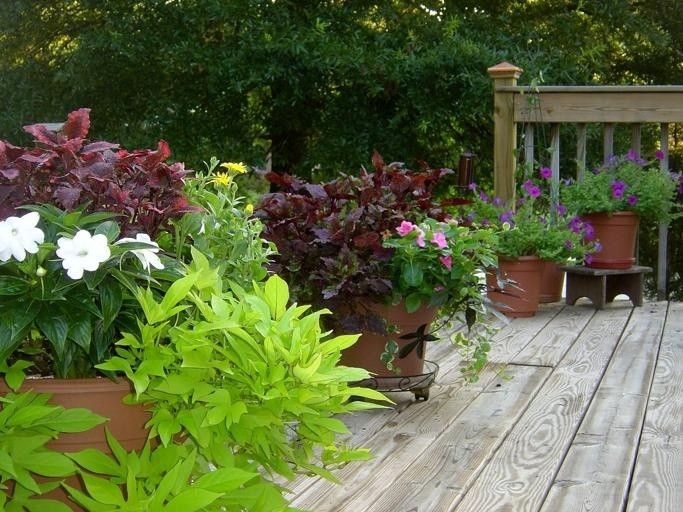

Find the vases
[[0, 376, 184, 512]]
